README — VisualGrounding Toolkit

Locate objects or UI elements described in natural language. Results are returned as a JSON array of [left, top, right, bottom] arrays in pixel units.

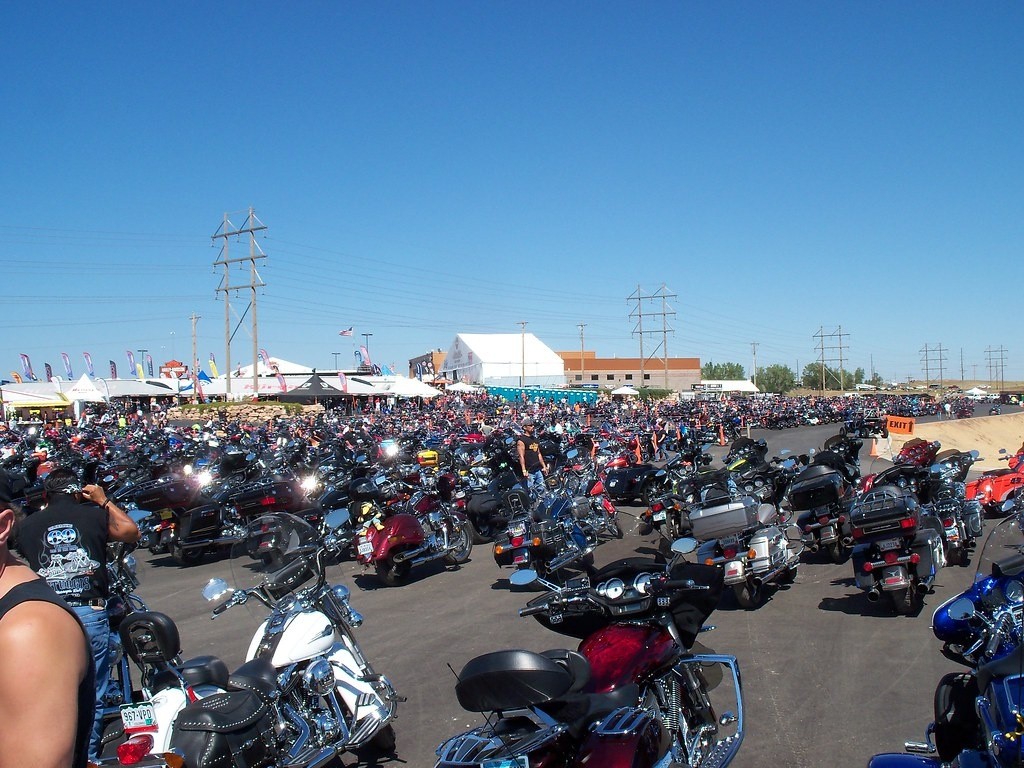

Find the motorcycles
[[101, 511, 184, 745], [867, 510, 1024, 768], [0, 396, 1024, 609], [431, 537, 746, 768], [117, 507, 408, 768]]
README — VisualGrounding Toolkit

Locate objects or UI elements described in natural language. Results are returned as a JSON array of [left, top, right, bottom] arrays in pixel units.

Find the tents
[[612, 387, 639, 397], [0, 373, 443, 418], [965, 388, 986, 401], [976, 383, 992, 390], [700, 380, 759, 392]]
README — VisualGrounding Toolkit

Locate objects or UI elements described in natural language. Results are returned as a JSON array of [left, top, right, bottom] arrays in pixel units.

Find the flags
[[340, 327, 353, 336]]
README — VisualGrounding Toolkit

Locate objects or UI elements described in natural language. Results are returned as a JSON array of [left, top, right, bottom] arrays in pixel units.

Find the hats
[[521, 418, 534, 429]]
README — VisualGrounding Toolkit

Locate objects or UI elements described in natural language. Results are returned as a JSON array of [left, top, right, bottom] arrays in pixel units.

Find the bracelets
[[100, 499, 111, 507]]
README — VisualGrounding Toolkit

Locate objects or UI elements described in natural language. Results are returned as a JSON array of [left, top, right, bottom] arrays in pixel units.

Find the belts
[[64, 598, 107, 608]]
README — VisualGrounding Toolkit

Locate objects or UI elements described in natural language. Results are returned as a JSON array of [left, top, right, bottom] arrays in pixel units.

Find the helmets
[[702, 453, 713, 465], [436, 473, 456, 492], [628, 438, 637, 451], [681, 448, 696, 461], [637, 519, 655, 536]]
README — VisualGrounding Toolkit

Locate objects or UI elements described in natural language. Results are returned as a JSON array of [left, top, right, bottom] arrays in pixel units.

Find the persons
[[0, 467, 96, 768], [0, 388, 967, 445], [518, 418, 549, 500], [14, 468, 141, 768]]
